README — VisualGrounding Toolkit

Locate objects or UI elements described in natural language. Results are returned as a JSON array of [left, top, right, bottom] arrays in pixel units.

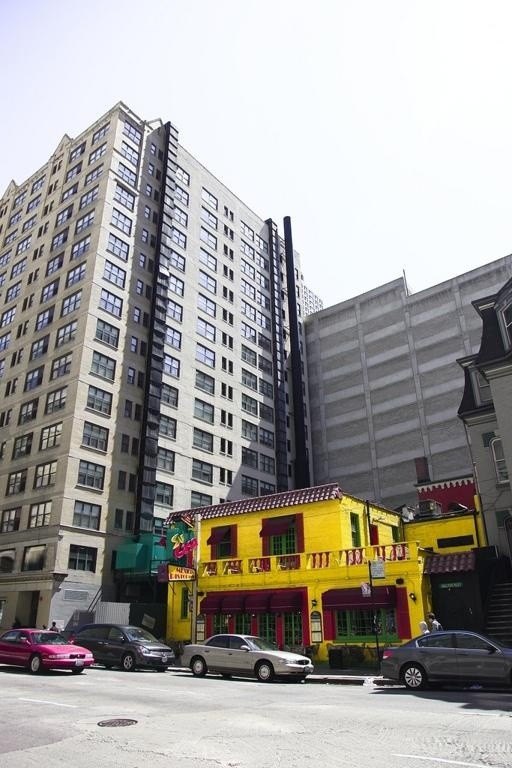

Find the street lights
[[171, 512, 201, 641]]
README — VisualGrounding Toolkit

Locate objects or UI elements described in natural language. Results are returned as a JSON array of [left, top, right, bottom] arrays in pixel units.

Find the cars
[[179, 633, 314, 683], [0, 629, 96, 678], [379, 630, 512, 691], [69, 623, 176, 673]]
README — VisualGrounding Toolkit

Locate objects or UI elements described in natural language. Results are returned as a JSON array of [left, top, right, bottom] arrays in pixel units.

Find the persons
[[13, 617, 22, 629], [49, 621, 60, 632], [43, 624, 49, 630], [426, 612, 443, 632], [419, 621, 429, 636]]
